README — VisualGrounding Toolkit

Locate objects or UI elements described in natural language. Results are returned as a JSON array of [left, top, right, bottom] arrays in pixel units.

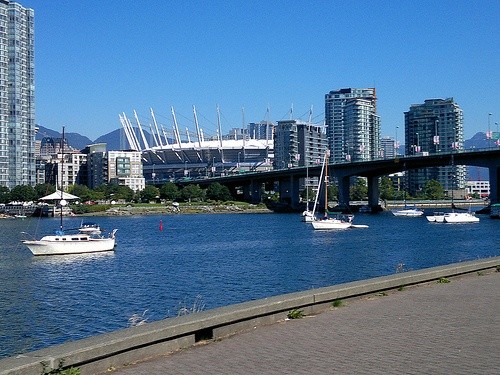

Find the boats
[[391, 207, 480, 222]]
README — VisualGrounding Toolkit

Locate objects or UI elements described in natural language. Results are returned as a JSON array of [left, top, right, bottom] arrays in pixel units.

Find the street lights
[[185, 161, 188, 178], [238, 152, 241, 170], [416, 133, 419, 153], [395, 126, 399, 157], [435, 120, 440, 152], [488, 113, 498, 147], [453, 128, 455, 150], [213, 157, 216, 177]]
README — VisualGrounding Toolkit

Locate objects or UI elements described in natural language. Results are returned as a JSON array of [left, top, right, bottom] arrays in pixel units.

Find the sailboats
[[302, 144, 369, 230], [19, 125, 119, 256]]
